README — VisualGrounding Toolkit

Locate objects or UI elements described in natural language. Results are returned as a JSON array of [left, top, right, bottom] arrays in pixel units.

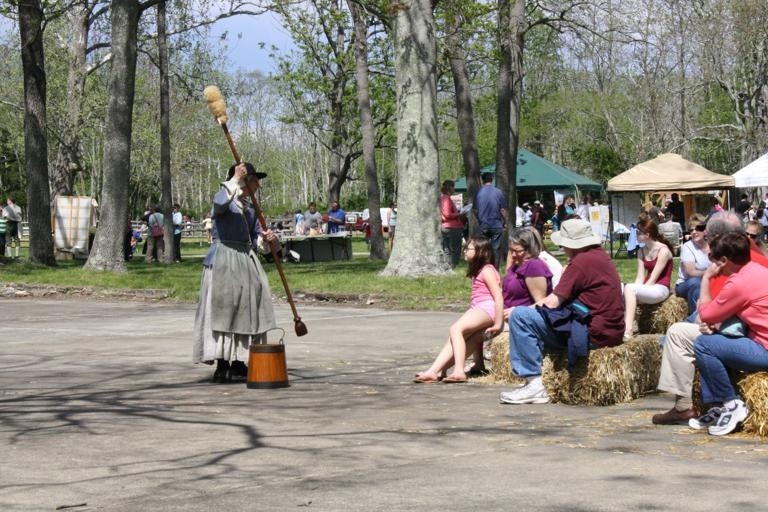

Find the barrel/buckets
[[246, 325, 289, 390]]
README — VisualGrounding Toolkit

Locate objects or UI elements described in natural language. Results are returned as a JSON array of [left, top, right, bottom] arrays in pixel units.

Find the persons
[[2, 198, 22, 259], [415, 193, 767, 436], [471, 172, 508, 272], [440, 179, 467, 268], [135, 204, 214, 261], [256, 200, 397, 249], [0, 206, 8, 267], [192, 162, 283, 382]]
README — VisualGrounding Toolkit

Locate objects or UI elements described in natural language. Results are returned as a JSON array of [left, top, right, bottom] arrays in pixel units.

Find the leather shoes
[[653, 406, 698, 425], [228, 361, 247, 383], [213, 359, 230, 384]]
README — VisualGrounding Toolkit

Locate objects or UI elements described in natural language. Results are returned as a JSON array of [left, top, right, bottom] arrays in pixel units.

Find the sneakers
[[689, 407, 721, 430], [708, 399, 750, 436], [500, 381, 550, 405]]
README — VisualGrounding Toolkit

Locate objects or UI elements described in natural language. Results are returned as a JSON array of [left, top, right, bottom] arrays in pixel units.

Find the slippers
[[442, 376, 467, 382], [412, 372, 438, 383]]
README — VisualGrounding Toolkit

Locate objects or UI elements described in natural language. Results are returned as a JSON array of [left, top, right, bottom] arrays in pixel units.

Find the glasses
[[746, 232, 757, 239], [635, 230, 643, 236], [689, 229, 694, 231], [707, 253, 713, 261], [464, 247, 474, 253]]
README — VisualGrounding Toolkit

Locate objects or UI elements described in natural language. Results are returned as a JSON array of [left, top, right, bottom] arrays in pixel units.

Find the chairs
[[658, 223, 685, 257]]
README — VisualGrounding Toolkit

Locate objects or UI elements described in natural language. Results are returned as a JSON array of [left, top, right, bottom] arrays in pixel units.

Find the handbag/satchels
[[151, 214, 163, 237]]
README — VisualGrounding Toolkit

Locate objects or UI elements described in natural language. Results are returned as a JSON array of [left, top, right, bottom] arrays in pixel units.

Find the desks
[[613, 230, 637, 259]]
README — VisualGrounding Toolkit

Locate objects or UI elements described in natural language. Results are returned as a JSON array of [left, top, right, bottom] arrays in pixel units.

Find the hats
[[226, 163, 267, 181], [550, 219, 601, 249], [695, 211, 716, 231]]
